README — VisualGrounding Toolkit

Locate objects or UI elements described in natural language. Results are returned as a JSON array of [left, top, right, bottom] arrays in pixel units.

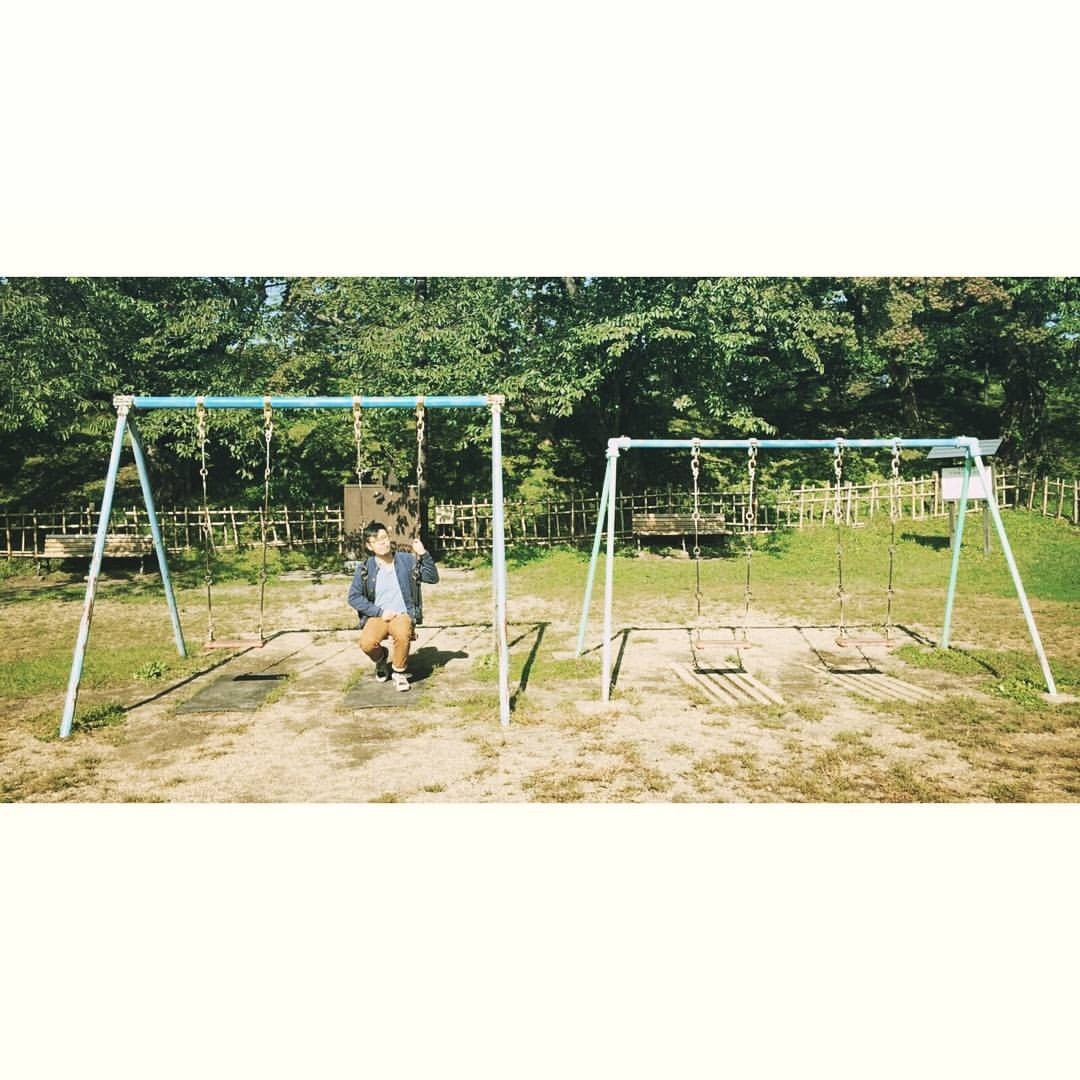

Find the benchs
[[33, 534, 153, 576], [632, 513, 734, 554]]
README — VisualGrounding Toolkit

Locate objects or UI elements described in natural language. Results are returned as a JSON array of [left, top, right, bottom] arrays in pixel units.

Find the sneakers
[[392, 672, 410, 691], [376, 647, 389, 683]]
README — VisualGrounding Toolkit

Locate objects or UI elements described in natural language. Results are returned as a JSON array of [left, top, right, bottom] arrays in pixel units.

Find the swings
[[352, 395, 425, 643], [194, 394, 272, 649], [832, 437, 902, 649], [690, 438, 756, 651]]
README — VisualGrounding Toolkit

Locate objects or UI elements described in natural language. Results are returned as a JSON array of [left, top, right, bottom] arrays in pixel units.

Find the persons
[[347, 522, 439, 690]]
